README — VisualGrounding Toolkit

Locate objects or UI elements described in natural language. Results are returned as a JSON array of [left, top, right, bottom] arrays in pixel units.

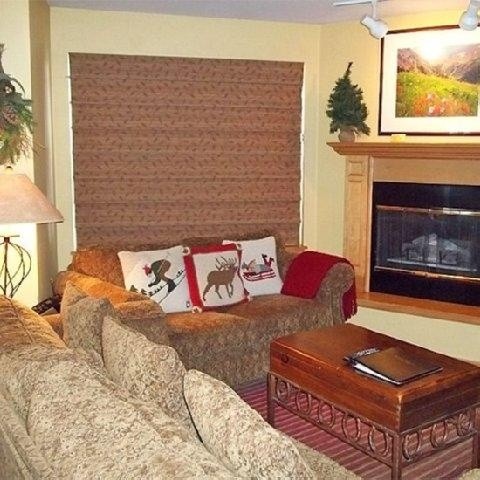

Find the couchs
[[51, 229, 356, 385], [0, 295, 365, 480]]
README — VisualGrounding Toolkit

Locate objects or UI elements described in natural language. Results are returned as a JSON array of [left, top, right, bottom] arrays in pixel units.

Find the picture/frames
[[377, 23, 480, 135]]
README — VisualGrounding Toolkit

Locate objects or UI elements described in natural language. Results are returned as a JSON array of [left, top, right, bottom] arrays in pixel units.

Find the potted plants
[[323, 59, 372, 143]]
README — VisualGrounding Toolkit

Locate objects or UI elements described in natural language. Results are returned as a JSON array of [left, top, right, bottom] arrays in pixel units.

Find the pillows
[[100, 314, 197, 447], [182, 242, 252, 315], [58, 278, 118, 363], [116, 245, 196, 315], [179, 367, 320, 479], [221, 236, 284, 297]]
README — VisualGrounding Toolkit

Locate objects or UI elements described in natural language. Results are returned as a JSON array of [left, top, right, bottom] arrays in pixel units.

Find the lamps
[[361, 3, 389, 39], [0, 163, 64, 295], [458, 0, 480, 30]]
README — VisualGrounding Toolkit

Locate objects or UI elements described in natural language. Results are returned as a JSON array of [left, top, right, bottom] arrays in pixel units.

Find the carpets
[[228, 368, 480, 479]]
